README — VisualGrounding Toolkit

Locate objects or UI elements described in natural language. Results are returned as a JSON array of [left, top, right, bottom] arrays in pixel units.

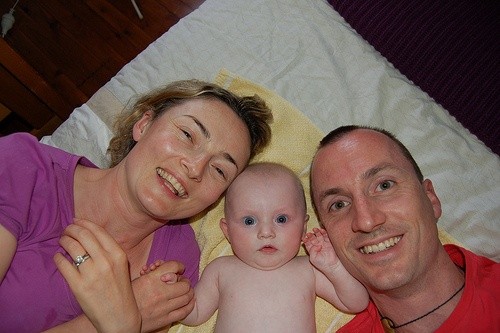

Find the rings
[[74, 252, 90, 269]]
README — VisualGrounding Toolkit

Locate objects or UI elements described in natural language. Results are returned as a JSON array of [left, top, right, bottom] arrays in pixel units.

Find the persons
[[309, 124, 500, 333], [0, 78, 275, 333], [140, 163, 370, 333]]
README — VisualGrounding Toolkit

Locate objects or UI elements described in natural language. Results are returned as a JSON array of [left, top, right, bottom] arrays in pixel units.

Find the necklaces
[[379, 263, 466, 333]]
